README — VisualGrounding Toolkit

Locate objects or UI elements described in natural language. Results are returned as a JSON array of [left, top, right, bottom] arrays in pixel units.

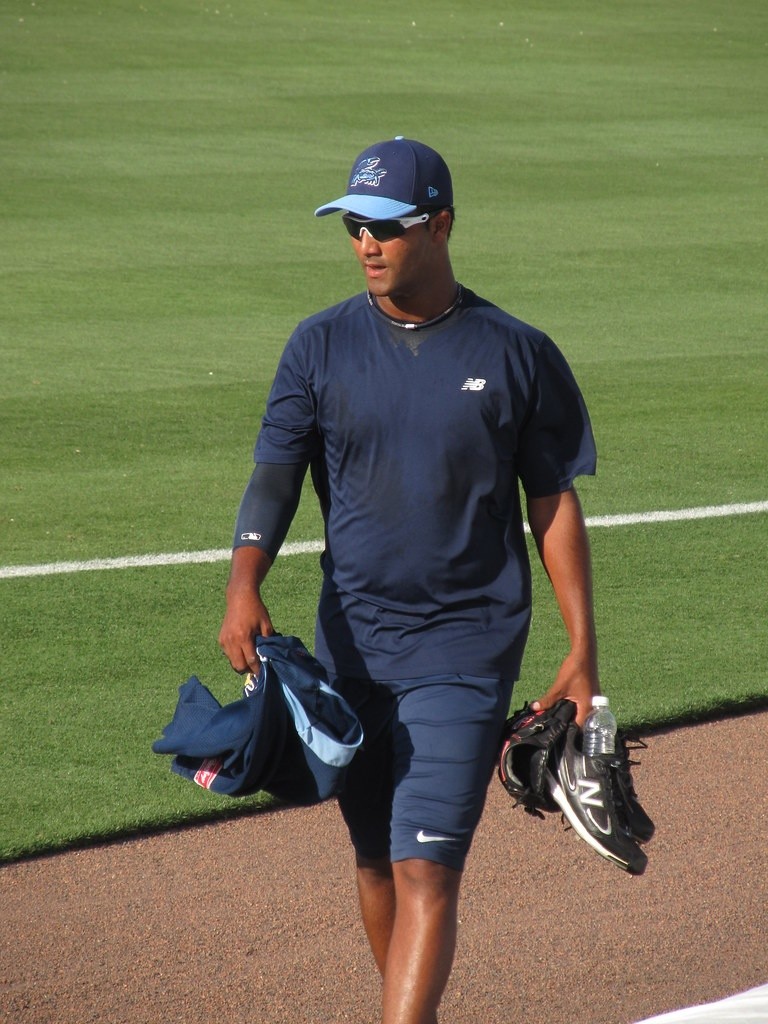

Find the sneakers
[[545, 722, 655, 875]]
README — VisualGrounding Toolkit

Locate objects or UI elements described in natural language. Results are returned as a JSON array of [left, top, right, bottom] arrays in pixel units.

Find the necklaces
[[367, 285, 461, 330]]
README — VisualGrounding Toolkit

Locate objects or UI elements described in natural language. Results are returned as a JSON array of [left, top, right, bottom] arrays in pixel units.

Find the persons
[[217, 140, 597, 1024]]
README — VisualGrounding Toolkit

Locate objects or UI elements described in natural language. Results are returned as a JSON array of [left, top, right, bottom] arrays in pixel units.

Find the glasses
[[342, 211, 439, 242]]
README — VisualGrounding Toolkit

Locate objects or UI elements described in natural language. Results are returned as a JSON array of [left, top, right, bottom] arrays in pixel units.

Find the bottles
[[583, 695, 617, 757]]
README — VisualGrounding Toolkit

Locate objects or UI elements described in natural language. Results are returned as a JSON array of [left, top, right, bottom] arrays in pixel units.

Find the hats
[[314, 136, 453, 219]]
